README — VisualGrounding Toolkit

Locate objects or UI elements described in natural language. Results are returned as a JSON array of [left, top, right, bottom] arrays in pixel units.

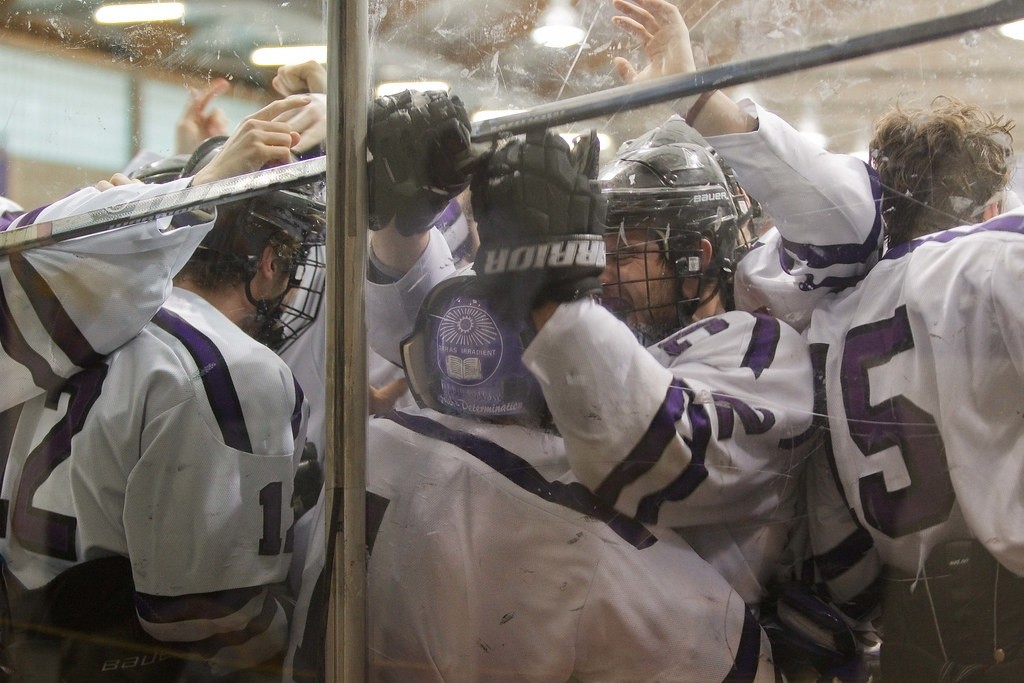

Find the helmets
[[173, 137, 328, 355], [600, 115, 760, 341], [399, 276, 553, 427]]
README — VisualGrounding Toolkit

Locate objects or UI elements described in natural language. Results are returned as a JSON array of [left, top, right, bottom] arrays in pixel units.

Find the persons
[[0, 0, 1024, 683]]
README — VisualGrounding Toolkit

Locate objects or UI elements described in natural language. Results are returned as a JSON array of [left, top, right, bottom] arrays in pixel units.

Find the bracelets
[[668, 88, 719, 127]]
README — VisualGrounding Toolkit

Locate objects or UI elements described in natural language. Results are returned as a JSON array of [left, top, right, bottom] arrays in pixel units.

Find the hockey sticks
[[0, 0, 1024, 254]]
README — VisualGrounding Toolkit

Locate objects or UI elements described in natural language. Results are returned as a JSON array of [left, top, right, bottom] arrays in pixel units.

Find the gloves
[[364, 92, 479, 236], [470, 128, 608, 305]]
